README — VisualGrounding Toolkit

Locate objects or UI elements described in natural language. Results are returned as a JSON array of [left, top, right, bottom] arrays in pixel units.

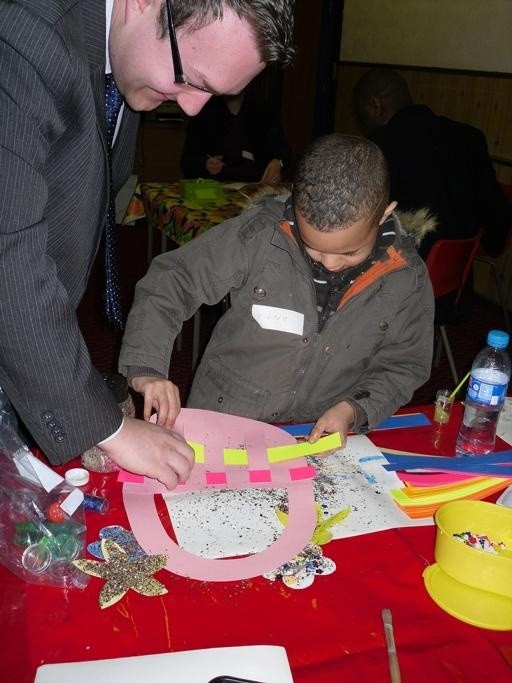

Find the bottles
[[79, 372, 137, 474], [454, 329, 512, 457], [433, 390, 455, 424]]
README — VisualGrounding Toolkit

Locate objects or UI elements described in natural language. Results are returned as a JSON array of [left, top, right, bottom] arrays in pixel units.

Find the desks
[[140, 181, 293, 373], [0, 394, 512, 683]]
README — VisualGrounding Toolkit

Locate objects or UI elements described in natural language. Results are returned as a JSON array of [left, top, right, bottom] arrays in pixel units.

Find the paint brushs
[[381, 608, 401, 683]]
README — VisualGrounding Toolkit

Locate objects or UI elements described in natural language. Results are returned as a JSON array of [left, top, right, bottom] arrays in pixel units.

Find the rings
[[272, 177, 276, 180]]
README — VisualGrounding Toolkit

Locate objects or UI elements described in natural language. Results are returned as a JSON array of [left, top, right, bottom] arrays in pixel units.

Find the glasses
[[165, 0, 216, 97]]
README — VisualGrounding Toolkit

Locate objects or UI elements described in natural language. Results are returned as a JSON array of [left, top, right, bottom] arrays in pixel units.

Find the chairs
[[471, 184, 512, 335], [424, 226, 485, 391]]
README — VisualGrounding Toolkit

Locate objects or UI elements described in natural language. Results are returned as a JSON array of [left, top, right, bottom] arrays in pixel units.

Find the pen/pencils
[[207, 153, 230, 170]]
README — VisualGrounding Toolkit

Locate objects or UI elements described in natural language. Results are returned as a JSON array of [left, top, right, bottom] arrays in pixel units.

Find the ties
[[101, 73, 127, 332]]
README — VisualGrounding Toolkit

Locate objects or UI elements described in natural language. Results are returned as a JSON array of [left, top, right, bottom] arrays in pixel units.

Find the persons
[[118, 132, 434, 460], [183, 87, 302, 188], [354, 70, 489, 258], [1, 2, 298, 491]]
